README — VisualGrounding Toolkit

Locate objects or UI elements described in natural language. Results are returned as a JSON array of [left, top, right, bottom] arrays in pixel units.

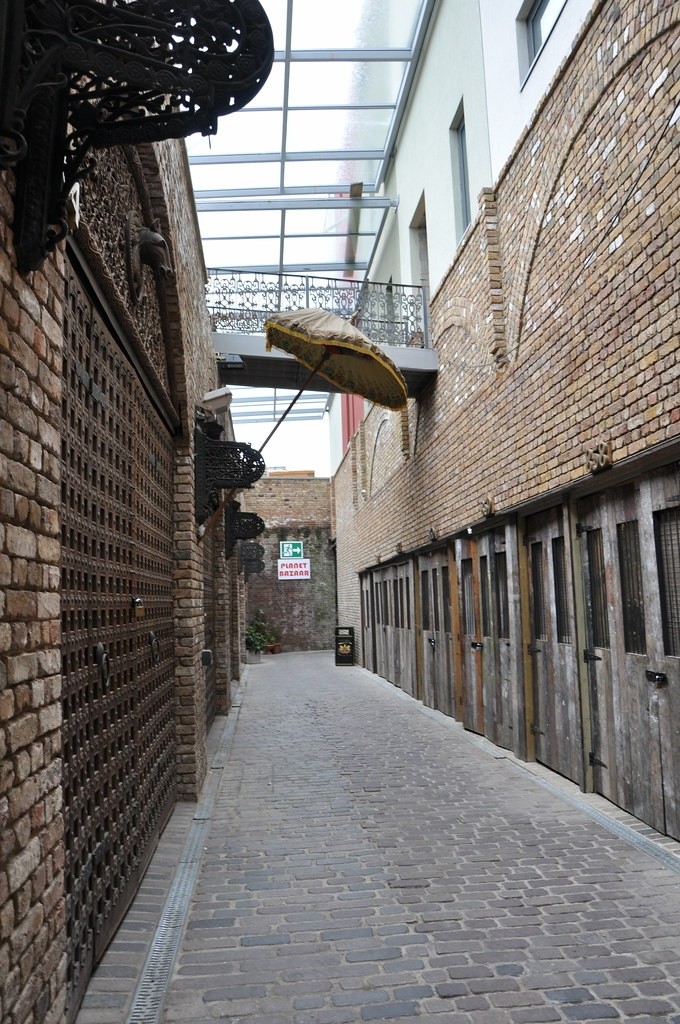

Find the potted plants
[[245, 609, 286, 664]]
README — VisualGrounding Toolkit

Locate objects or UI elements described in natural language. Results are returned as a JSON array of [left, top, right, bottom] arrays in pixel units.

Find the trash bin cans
[[335, 626, 355, 666]]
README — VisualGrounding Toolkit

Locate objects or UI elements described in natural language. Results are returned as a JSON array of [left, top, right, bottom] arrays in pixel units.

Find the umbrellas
[[199, 307, 408, 544]]
